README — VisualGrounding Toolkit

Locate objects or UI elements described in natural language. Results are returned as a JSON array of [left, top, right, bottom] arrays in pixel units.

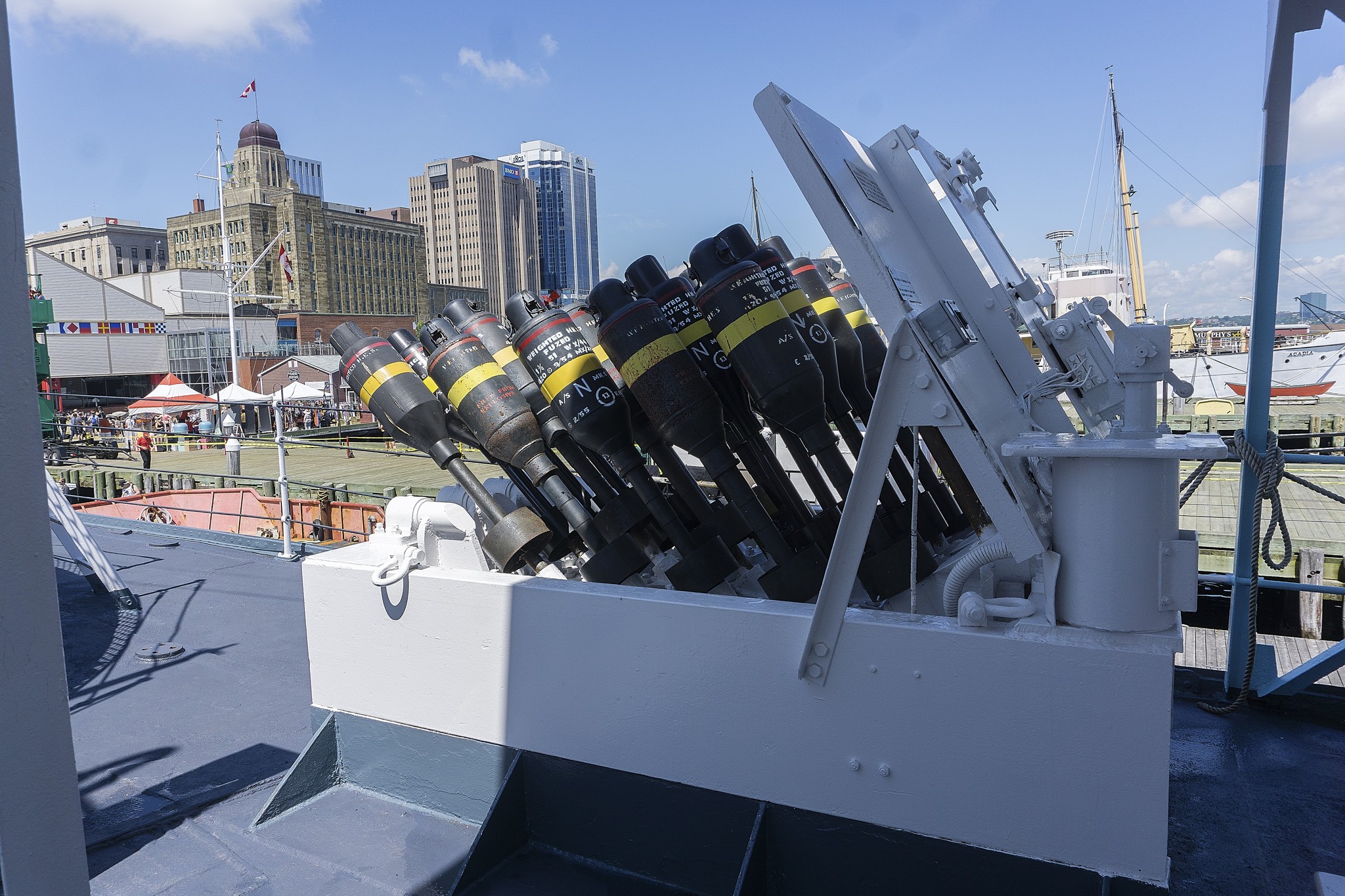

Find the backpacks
[[91, 415, 97, 424]]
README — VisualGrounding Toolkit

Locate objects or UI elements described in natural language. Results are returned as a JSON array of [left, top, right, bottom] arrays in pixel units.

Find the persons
[[28, 285, 45, 300], [59, 408, 111, 441], [119, 480, 140, 497], [59, 478, 72, 504], [323, 410, 330, 427], [241, 409, 246, 428], [155, 413, 178, 432], [302, 409, 311, 430], [115, 414, 136, 440], [137, 432, 153, 471], [377, 420, 398, 452], [185, 416, 202, 440]]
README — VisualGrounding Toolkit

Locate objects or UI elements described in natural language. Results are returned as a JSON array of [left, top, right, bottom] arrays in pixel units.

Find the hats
[[73, 409, 78, 413], [303, 409, 311, 414], [143, 432, 150, 437], [77, 412, 83, 417], [85, 421, 91, 425]]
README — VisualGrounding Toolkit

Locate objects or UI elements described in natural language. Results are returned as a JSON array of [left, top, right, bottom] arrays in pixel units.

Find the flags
[[277, 244, 292, 282]]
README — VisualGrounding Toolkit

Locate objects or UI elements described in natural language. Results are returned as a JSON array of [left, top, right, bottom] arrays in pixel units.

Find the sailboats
[[995, 63, 1345, 398]]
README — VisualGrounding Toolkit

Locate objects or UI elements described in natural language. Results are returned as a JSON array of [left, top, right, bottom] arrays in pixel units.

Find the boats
[[1273, 297, 1345, 352], [1223, 379, 1336, 399]]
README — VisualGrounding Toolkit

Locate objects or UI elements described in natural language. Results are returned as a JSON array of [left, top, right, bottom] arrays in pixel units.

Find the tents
[[207, 383, 277, 419], [128, 373, 220, 444], [268, 381, 335, 408]]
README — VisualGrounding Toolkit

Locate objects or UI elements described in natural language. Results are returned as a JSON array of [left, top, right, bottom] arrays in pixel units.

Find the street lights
[[323, 384, 342, 446], [273, 383, 286, 432], [203, 387, 223, 435]]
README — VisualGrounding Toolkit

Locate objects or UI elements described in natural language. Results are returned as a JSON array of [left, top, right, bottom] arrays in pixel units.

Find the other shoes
[[64, 438, 67, 440], [69, 440, 72, 442], [93, 436, 95, 437], [98, 436, 100, 437]]
